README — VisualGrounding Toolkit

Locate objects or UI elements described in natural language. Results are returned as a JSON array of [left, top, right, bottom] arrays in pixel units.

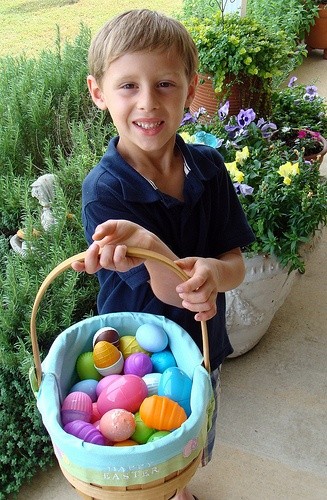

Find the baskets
[[27, 247, 215, 500]]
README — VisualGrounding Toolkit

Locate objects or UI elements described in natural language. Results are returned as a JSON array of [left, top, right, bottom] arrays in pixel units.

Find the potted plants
[[179, 9, 308, 128]]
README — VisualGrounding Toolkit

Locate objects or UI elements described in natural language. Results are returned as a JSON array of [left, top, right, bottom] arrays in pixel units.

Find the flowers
[[267, 75, 327, 156], [176, 124, 327, 278]]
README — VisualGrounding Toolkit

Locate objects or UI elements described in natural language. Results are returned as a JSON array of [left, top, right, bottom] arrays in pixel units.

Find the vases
[[268, 126, 327, 174], [224, 247, 298, 359]]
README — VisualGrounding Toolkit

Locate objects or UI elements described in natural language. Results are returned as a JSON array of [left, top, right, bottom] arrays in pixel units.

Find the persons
[[68, 8, 253, 500]]
